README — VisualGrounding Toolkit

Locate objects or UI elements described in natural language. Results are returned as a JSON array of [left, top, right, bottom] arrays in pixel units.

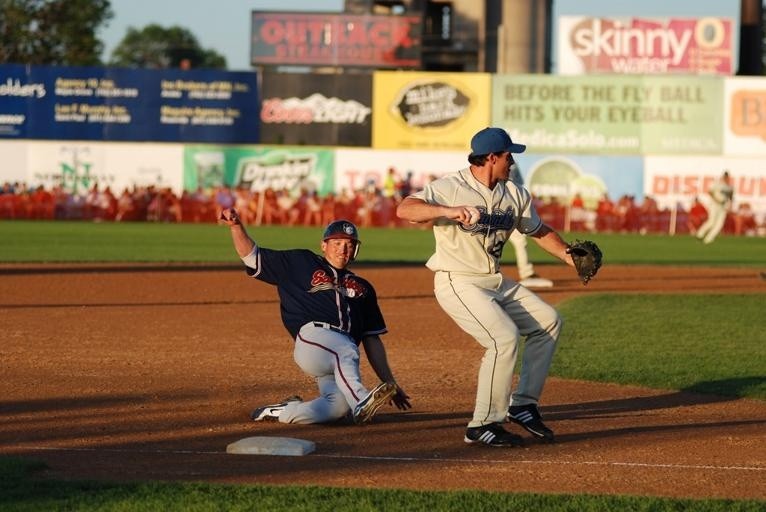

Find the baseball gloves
[[565, 238, 602, 287]]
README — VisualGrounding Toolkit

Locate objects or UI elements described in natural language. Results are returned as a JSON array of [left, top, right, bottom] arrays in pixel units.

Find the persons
[[217, 208, 413, 425], [396, 126, 604, 447], [505, 164, 554, 288]]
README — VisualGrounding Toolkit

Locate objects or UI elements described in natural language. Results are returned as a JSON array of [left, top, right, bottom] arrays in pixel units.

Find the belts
[[314, 322, 352, 339]]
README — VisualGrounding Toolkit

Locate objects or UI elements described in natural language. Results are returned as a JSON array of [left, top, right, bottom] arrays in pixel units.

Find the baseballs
[[469, 210, 477, 225]]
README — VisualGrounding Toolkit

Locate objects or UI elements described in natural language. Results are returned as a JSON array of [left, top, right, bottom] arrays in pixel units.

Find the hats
[[321, 219, 362, 244], [470, 126, 527, 157]]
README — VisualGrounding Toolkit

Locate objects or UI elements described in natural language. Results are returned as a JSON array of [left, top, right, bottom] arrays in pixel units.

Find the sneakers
[[463, 421, 524, 448], [506, 402, 554, 442], [351, 381, 398, 426], [249, 395, 304, 423]]
